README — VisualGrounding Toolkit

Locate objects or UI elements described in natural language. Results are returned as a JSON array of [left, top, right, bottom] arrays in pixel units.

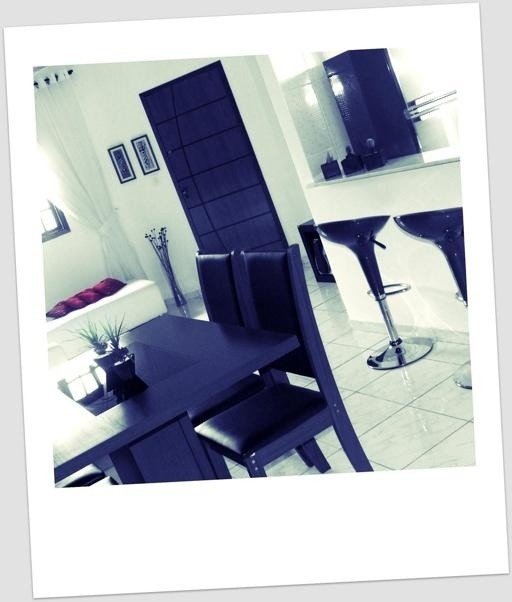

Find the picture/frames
[[107, 144, 136, 184], [131, 135, 160, 176]]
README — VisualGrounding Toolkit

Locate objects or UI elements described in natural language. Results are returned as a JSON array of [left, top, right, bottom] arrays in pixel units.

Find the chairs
[[183, 249, 265, 480], [194, 243, 374, 476]]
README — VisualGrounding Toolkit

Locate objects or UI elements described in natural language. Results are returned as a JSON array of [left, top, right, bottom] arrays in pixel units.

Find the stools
[[313, 215, 434, 369], [394, 208, 473, 390], [47, 278, 167, 369]]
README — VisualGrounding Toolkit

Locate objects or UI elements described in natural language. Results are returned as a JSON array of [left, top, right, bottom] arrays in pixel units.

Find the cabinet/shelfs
[[323, 48, 421, 161]]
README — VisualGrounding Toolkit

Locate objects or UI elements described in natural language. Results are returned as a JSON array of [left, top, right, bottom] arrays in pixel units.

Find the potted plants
[[95, 313, 135, 381], [63, 317, 114, 374]]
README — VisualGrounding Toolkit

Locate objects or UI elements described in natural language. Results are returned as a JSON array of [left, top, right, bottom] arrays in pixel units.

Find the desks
[[49, 314, 301, 486]]
